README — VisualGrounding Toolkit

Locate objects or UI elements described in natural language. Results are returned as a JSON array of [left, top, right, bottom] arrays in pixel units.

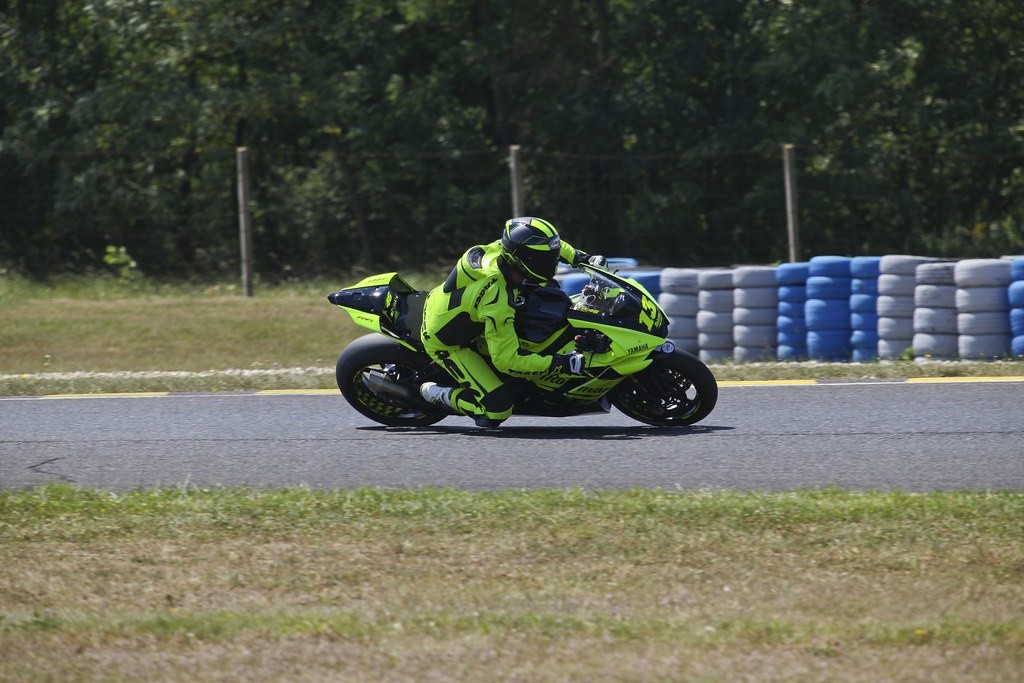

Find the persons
[[419, 216, 609, 431]]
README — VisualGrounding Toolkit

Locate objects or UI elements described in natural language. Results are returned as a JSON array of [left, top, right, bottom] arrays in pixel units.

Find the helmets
[[500, 215, 562, 290]]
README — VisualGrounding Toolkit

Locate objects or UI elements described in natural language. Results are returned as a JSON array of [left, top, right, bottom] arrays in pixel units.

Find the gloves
[[547, 350, 586, 376], [573, 250, 609, 274]]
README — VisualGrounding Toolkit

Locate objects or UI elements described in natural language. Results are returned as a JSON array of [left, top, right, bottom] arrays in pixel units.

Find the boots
[[419, 382, 455, 410]]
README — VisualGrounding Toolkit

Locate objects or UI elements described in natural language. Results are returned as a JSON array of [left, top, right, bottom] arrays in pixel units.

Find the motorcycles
[[326, 259, 719, 429]]
